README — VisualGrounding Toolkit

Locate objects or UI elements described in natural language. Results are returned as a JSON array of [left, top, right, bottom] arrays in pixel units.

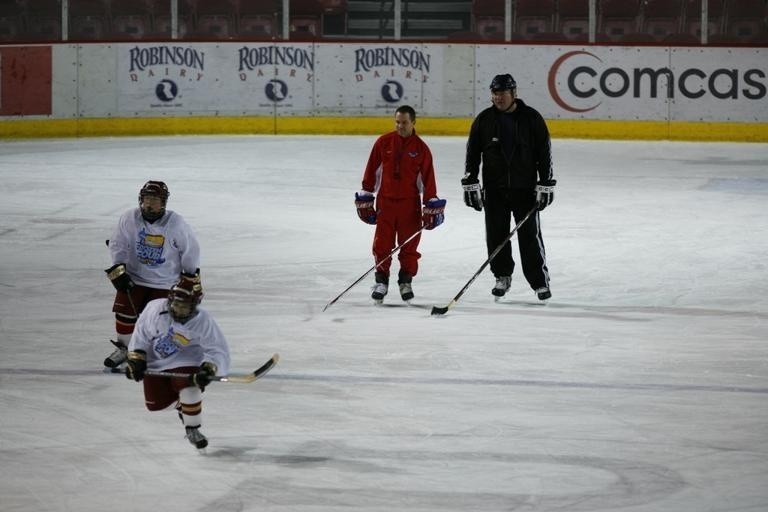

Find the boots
[[183, 422, 208, 449], [398, 272, 414, 300], [104, 339, 128, 367], [371, 272, 389, 300]]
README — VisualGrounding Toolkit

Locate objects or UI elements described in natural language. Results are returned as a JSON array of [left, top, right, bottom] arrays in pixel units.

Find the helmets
[[138, 180, 170, 223], [167, 281, 201, 324], [489, 74, 516, 91]]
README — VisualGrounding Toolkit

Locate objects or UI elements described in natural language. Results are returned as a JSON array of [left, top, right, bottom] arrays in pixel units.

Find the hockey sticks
[[322, 224, 429, 311], [108, 353, 278, 385], [432, 201, 539, 315]]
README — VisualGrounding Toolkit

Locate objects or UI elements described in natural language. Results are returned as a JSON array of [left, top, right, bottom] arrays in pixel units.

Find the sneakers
[[534, 286, 551, 299], [492, 275, 511, 296]]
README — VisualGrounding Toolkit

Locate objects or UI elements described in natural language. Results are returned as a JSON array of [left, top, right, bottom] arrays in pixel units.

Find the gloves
[[461, 177, 486, 211], [125, 349, 148, 381], [422, 198, 446, 230], [181, 268, 203, 303], [533, 179, 557, 211], [187, 362, 217, 392], [104, 263, 135, 297], [355, 192, 378, 225]]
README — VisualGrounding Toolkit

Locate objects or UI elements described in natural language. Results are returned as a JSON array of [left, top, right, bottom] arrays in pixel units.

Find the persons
[[354, 106, 447, 299], [100, 177, 203, 370], [463, 74, 558, 303], [123, 278, 230, 448]]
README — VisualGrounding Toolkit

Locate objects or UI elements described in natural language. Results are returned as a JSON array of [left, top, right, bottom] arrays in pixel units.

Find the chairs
[[0, 1, 351, 43], [441, 0, 768, 44]]
